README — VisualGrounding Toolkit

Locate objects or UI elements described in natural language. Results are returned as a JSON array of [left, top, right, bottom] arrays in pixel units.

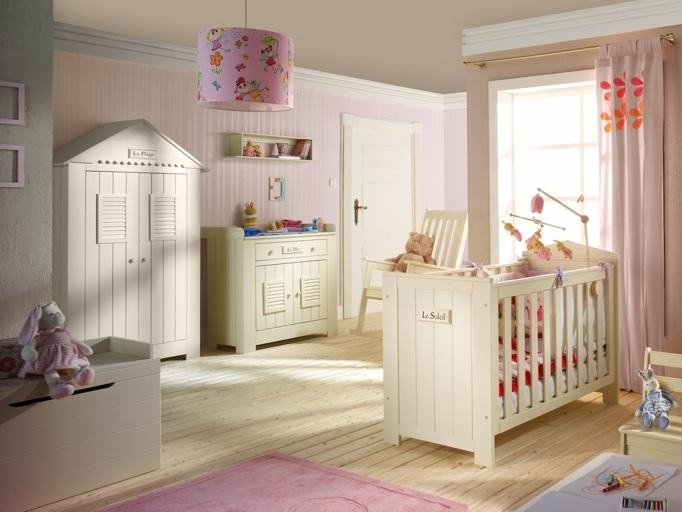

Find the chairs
[[618, 347, 682, 457], [355, 209, 468, 318]]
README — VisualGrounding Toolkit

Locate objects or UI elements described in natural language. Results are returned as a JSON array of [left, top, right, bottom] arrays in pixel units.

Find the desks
[[513, 451, 682, 511]]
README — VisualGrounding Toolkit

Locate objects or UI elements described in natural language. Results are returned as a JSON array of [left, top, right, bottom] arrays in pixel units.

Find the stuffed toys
[[384, 231, 438, 273], [498, 302, 517, 345], [524, 305, 543, 338], [15, 299, 95, 400], [243, 200, 258, 229], [634, 368, 681, 431]]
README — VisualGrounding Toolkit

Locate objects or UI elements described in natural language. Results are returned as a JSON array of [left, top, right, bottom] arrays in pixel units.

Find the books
[[278, 139, 313, 160]]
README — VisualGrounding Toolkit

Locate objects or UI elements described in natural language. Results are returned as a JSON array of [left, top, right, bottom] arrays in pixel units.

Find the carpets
[[94, 452, 467, 512]]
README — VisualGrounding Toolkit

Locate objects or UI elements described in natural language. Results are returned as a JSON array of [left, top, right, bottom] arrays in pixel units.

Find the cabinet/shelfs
[[200, 223, 338, 353], [53, 119, 209, 362], [0, 337, 161, 512]]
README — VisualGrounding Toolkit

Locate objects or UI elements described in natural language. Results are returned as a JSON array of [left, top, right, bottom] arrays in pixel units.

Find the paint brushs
[[623, 498, 664, 510], [602, 474, 625, 492]]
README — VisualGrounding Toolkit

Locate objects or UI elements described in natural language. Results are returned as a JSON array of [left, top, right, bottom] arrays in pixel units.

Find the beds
[[382, 241, 618, 466]]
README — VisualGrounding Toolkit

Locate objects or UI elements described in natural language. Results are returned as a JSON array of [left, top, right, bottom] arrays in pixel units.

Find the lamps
[[197, 0, 294, 112]]
